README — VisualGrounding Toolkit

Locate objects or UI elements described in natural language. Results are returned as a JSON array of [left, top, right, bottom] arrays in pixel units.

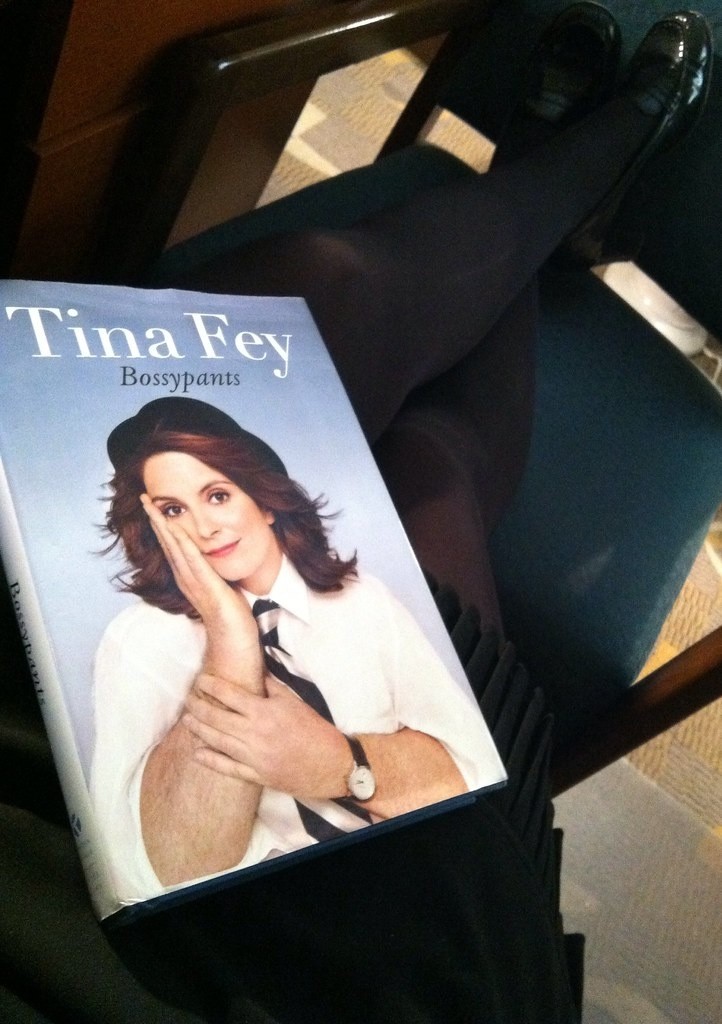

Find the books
[[0, 279, 508, 930]]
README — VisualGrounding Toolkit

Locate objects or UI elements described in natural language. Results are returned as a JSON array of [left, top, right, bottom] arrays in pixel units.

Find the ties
[[251, 600, 374, 842]]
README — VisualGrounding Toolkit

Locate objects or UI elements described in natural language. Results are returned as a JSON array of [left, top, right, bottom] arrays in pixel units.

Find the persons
[[0, 0, 715, 1024], [89, 396, 507, 896]]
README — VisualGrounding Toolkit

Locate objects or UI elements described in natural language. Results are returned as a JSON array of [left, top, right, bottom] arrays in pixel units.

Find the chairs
[[74, 0, 722, 796]]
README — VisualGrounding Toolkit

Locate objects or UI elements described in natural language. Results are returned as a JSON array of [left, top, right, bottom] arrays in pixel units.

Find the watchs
[[342, 733, 375, 802]]
